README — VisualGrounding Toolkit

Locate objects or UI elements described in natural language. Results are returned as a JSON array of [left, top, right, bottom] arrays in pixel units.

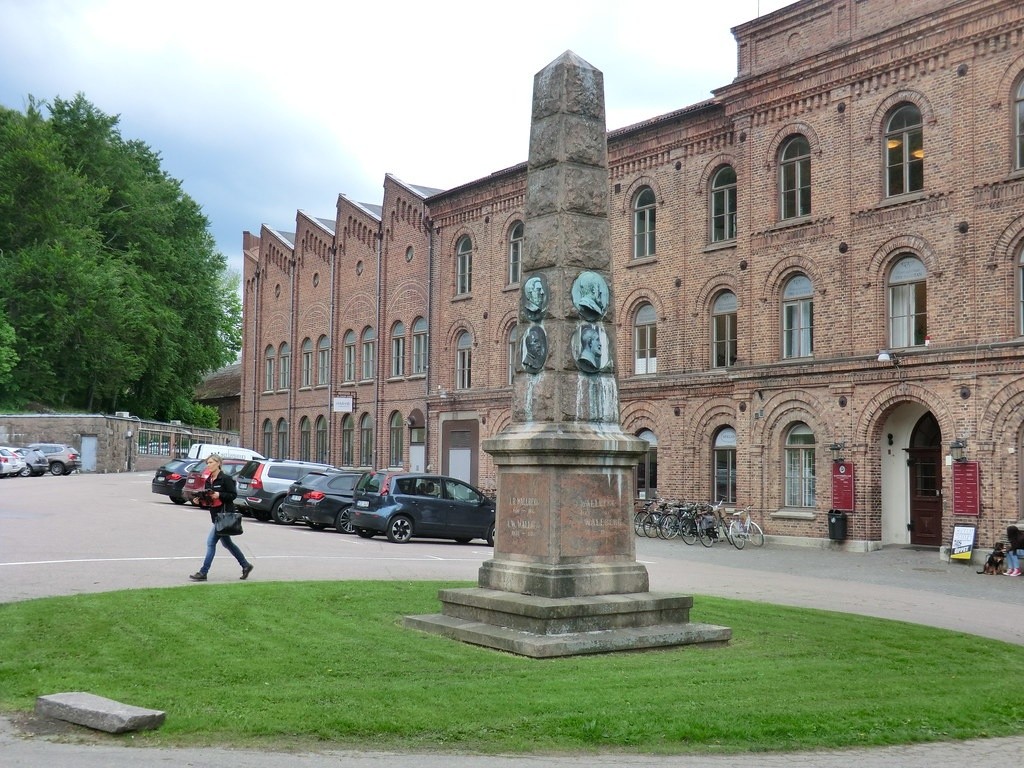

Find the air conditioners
[[170, 420, 181, 426], [115, 412, 129, 418]]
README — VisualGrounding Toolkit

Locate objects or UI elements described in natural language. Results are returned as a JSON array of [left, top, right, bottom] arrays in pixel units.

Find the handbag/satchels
[[214, 502, 244, 536]]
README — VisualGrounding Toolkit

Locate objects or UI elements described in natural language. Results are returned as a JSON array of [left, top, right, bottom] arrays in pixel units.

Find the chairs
[[418, 483, 436, 496]]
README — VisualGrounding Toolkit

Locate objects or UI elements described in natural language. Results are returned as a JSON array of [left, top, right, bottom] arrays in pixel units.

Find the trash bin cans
[[828, 509, 848, 540]]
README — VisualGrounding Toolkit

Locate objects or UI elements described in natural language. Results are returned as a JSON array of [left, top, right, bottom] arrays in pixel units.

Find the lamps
[[878, 351, 898, 364], [440, 391, 455, 400]]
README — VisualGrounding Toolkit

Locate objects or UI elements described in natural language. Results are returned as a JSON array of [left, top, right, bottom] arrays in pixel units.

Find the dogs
[[976, 542, 1005, 575]]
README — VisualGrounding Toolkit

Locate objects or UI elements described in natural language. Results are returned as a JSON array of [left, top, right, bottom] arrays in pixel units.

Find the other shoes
[[1003, 568, 1013, 575], [1010, 568, 1022, 576]]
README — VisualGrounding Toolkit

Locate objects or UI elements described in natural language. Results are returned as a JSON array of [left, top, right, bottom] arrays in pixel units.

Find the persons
[[1002, 526, 1024, 577], [577, 274, 604, 369], [522, 276, 544, 370], [189, 454, 254, 580]]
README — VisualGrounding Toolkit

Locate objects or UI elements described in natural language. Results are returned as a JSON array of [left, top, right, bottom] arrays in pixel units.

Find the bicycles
[[729, 503, 765, 550], [630, 496, 732, 549]]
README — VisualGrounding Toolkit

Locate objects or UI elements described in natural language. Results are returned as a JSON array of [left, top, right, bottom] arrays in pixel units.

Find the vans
[[187, 443, 266, 462]]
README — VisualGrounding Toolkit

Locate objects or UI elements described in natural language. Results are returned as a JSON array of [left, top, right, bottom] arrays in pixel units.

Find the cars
[[280, 467, 434, 534]]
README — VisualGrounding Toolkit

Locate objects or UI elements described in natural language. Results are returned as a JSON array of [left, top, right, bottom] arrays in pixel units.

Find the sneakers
[[239, 562, 254, 579], [188, 572, 208, 582]]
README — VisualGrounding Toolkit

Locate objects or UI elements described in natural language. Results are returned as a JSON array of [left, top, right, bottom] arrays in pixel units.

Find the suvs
[[28, 443, 83, 476], [0, 447, 27, 478], [14, 447, 50, 477], [181, 457, 249, 510], [151, 457, 207, 505], [348, 471, 496, 547], [236, 456, 345, 526]]
